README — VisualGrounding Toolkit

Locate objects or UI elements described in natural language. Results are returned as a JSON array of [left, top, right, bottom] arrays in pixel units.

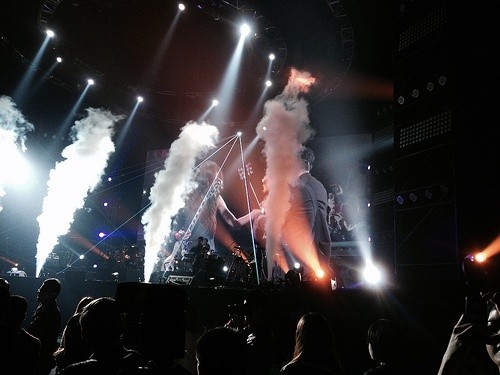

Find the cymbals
[[233, 246, 245, 250]]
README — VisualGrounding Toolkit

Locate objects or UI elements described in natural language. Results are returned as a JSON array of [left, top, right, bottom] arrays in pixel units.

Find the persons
[[282, 146, 331, 275], [189, 236, 210, 254], [170, 160, 263, 251], [0, 276, 500, 375]]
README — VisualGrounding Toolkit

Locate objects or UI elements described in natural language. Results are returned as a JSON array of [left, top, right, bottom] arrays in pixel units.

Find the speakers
[[116, 282, 186, 360]]
